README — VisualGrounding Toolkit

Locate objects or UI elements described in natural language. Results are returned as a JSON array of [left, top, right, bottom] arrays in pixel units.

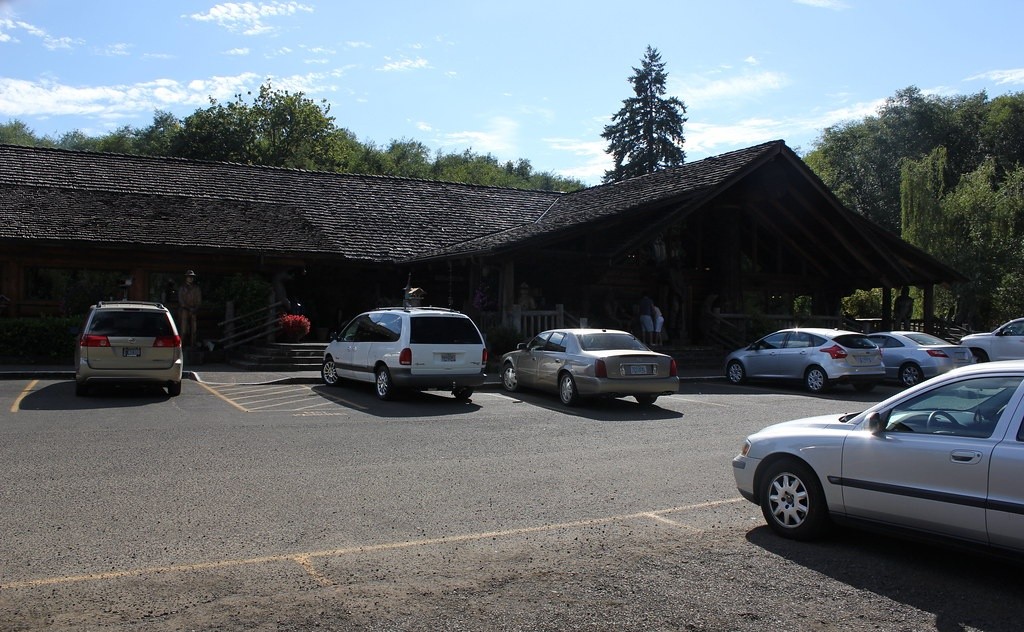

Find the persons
[[179, 269, 202, 347], [601, 291, 631, 329], [653, 303, 664, 345], [893, 286, 913, 331], [638, 291, 655, 346]]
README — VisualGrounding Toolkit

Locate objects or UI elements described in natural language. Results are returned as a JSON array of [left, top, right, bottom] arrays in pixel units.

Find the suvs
[[72, 300, 183, 397], [321, 306, 488, 401]]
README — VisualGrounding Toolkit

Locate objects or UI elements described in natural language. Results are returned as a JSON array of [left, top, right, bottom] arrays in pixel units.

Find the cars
[[730, 360, 1024, 568], [722, 327, 887, 395], [959, 318, 1024, 363], [498, 328, 679, 408], [863, 330, 977, 389]]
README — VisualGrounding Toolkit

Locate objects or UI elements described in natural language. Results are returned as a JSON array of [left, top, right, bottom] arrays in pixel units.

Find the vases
[[288, 332, 302, 344]]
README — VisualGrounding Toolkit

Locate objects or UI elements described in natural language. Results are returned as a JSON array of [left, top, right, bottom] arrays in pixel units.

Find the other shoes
[[650, 344, 655, 346]]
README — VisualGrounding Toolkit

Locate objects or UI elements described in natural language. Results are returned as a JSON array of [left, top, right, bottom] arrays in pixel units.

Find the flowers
[[280, 313, 312, 337]]
